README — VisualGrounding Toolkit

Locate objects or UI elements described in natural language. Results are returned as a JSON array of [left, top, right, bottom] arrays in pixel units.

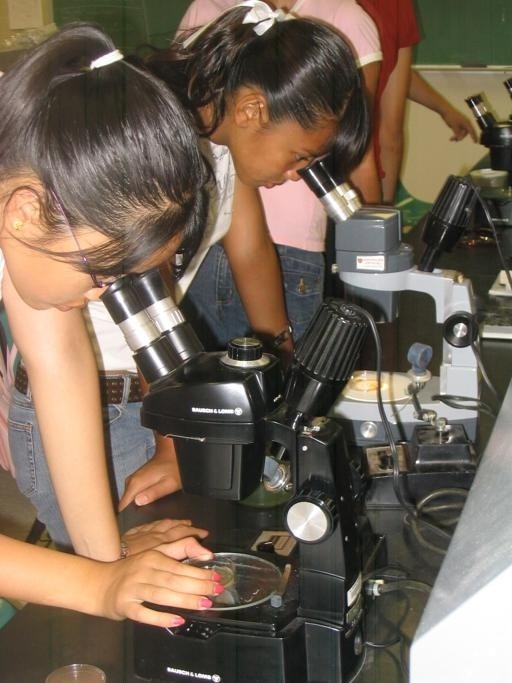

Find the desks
[[0, 211, 512, 683]]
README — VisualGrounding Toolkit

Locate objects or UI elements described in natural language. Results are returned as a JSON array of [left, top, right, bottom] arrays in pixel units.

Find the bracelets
[[261, 322, 295, 353], [116, 536, 130, 561]]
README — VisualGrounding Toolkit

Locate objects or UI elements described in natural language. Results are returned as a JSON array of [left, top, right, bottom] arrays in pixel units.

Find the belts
[[12, 362, 145, 403]]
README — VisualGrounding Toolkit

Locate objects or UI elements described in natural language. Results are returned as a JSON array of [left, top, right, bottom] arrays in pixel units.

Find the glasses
[[48, 188, 121, 289]]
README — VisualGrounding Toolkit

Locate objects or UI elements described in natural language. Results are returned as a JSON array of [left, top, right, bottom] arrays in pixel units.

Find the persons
[[349, 0, 425, 216], [170, 0, 386, 369], [1, 0, 374, 556], [404, 67, 481, 144], [0, 21, 225, 631]]
